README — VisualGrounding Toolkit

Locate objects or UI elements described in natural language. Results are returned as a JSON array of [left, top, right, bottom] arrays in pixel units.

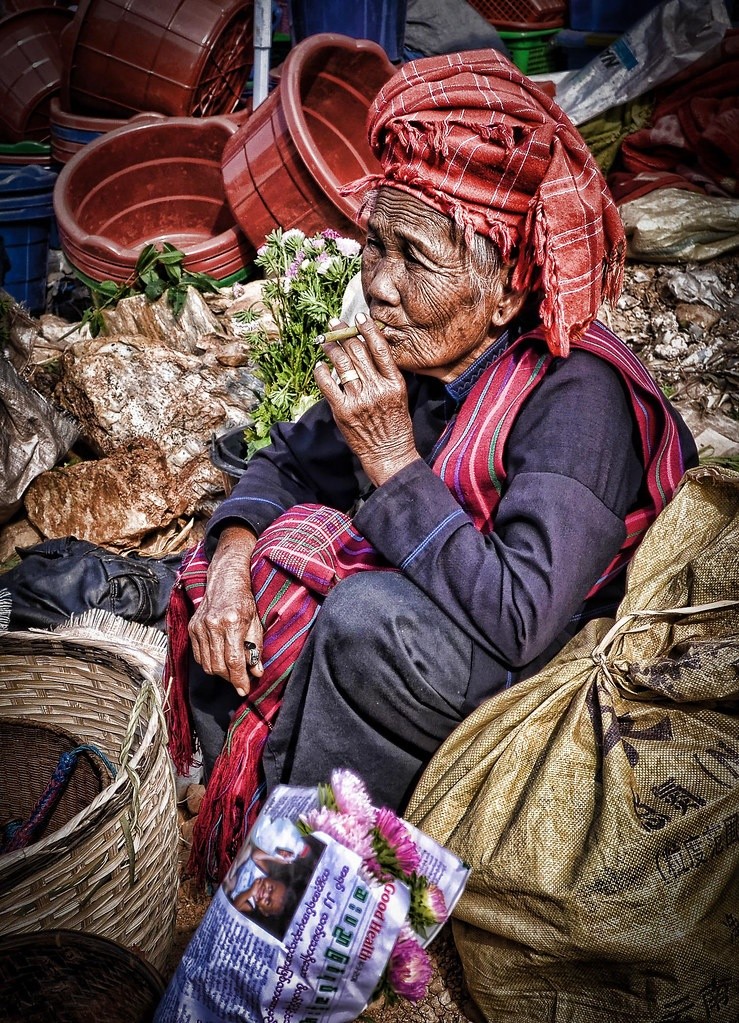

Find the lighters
[[244, 641, 260, 666]]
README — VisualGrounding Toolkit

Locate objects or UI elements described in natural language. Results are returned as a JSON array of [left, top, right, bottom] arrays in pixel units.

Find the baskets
[[467, 0, 566, 75], [0, 631, 176, 1023]]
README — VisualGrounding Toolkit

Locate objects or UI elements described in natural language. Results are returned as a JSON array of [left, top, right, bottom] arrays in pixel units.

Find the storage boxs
[[467, 0, 566, 76]]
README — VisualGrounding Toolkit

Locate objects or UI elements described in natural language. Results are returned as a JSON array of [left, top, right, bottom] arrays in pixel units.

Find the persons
[[224, 846, 297, 918], [164, 50, 697, 883]]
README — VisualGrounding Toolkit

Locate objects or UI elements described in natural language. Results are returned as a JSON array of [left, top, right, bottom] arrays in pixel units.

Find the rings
[[339, 370, 359, 385]]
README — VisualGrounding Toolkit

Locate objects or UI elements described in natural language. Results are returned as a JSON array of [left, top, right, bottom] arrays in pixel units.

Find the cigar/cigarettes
[[314, 319, 386, 345]]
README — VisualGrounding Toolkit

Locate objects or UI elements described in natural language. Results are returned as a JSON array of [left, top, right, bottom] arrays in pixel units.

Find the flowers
[[232, 227, 363, 461], [297, 767, 448, 1010]]
